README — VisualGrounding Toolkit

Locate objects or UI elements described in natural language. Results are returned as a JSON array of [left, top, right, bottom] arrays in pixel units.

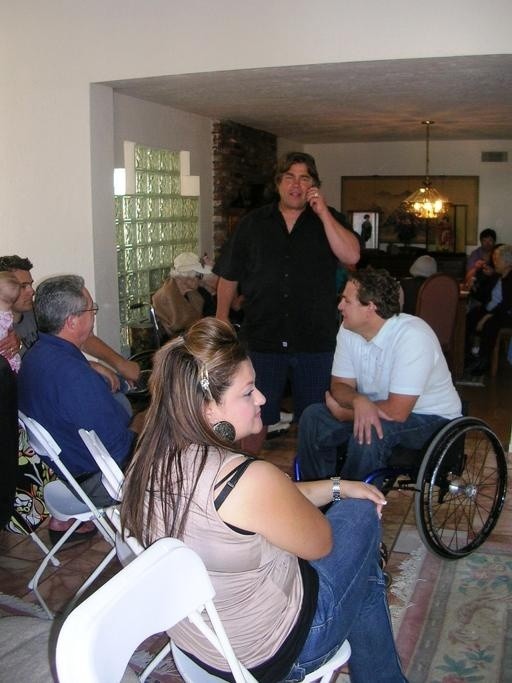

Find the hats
[[174, 253, 213, 273]]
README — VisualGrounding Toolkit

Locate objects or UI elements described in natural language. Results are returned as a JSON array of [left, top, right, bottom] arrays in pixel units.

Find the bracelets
[[330, 474, 342, 504]]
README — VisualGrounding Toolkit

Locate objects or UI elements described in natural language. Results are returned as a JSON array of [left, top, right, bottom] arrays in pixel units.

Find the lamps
[[402, 135, 450, 219]]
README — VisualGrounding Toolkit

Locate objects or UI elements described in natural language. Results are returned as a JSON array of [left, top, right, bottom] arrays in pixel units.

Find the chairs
[[363, 244, 511, 394]]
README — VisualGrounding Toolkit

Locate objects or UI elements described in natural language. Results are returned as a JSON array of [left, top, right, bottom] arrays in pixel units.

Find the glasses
[[82, 303, 98, 314]]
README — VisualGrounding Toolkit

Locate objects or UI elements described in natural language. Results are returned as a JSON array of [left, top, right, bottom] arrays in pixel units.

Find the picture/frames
[[341, 175, 479, 245]]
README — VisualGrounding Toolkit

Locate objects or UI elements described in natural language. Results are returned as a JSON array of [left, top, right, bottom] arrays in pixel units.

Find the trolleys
[[292, 415, 507, 586], [123, 301, 167, 402]]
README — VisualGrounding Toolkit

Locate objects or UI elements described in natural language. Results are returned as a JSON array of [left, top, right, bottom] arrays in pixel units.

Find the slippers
[[49, 518, 97, 543]]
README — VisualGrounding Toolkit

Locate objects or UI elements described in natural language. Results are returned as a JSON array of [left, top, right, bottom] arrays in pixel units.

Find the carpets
[[388, 536, 512, 683]]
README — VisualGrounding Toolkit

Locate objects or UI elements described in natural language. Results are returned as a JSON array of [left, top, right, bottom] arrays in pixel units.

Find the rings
[[314, 193, 318, 197]]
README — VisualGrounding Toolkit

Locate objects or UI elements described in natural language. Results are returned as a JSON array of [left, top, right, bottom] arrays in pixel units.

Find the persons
[[296, 267, 466, 483], [361, 214, 373, 247], [210, 149, 365, 458], [118, 315, 410, 683], [151, 251, 244, 333], [436, 214, 453, 251], [1, 255, 148, 545], [392, 227, 511, 388]]
